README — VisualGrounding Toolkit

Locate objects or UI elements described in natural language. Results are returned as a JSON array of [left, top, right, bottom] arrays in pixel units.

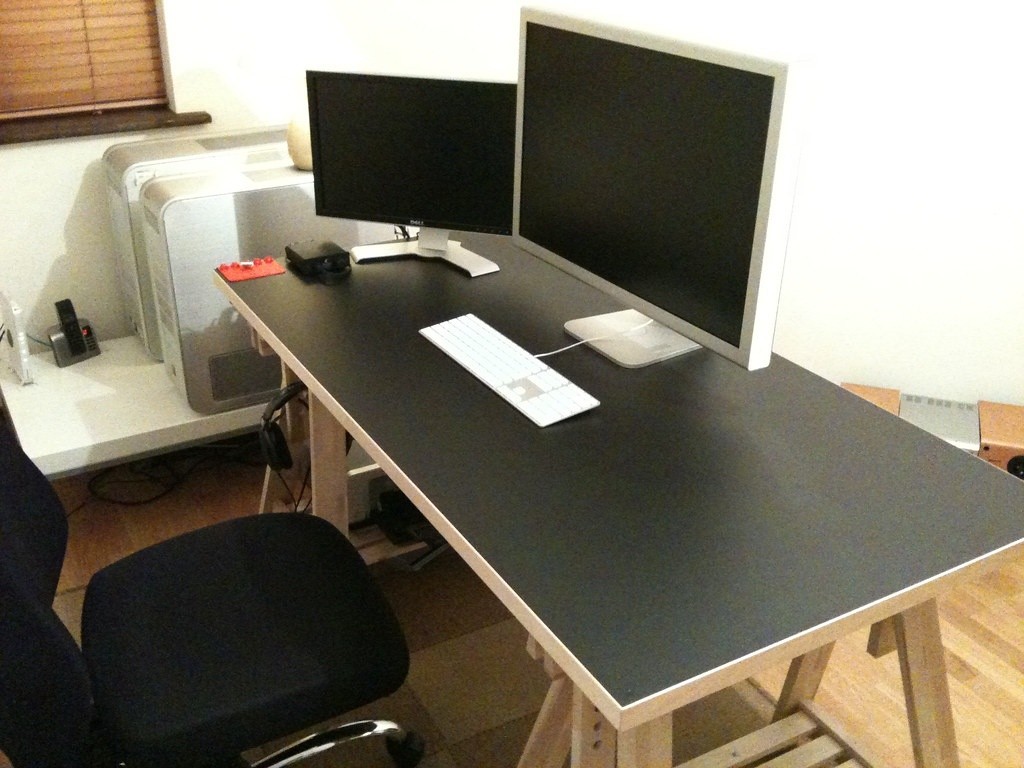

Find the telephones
[[46, 298, 102, 368]]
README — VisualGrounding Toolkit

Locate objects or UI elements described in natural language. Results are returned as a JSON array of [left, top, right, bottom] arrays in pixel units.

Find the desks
[[0, 333, 280, 484], [212, 233, 1024, 768]]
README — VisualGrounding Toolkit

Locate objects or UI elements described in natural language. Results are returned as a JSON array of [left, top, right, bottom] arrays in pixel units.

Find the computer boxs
[[105, 125, 398, 414]]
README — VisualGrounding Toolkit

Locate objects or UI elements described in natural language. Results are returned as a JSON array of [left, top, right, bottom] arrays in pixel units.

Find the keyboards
[[419, 313, 600, 427]]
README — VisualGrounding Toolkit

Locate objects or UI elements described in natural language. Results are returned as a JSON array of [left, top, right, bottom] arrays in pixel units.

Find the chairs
[[0, 390, 430, 768]]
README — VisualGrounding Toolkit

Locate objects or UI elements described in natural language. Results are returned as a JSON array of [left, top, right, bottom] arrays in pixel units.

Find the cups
[[288, 119, 312, 170]]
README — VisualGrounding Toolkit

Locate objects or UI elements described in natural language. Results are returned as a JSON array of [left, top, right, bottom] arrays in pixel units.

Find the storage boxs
[[899, 394, 980, 456]]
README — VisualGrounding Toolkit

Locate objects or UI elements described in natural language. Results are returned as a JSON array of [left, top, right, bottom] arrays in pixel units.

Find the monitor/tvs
[[519, 7, 798, 371], [307, 70, 518, 277]]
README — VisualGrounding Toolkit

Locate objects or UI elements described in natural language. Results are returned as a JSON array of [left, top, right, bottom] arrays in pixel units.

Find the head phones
[[258, 380, 353, 470]]
